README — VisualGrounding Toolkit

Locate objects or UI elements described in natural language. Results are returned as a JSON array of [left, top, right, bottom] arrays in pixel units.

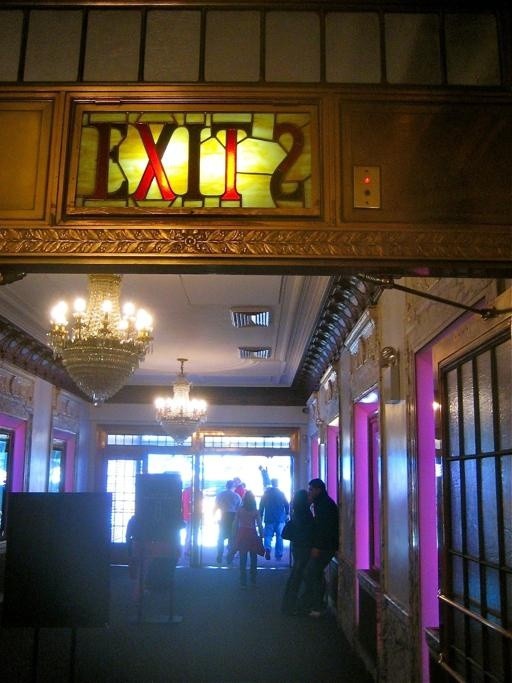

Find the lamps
[[341, 272, 493, 321], [154, 358, 209, 444], [47, 274, 155, 408]]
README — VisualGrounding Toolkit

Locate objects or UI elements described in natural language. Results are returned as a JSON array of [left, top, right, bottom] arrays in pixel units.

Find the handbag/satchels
[[281, 513, 295, 541]]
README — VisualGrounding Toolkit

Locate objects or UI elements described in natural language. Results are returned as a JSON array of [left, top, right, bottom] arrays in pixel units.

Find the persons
[[301, 477, 340, 617], [280, 488, 314, 614], [181, 465, 289, 591], [126, 514, 138, 578]]
